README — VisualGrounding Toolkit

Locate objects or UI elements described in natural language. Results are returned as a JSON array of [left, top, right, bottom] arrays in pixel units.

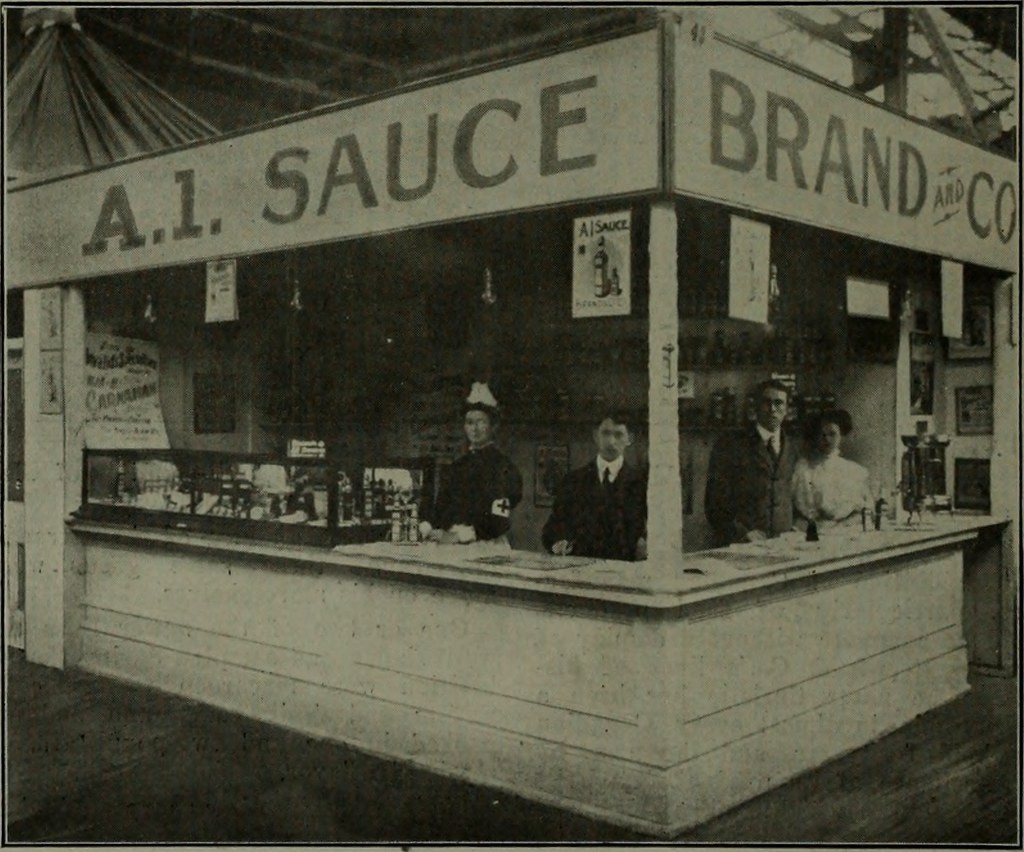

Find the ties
[[602, 466, 612, 495], [768, 438, 780, 466]]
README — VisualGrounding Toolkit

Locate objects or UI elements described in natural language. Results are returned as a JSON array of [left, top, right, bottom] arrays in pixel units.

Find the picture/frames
[[433, 446, 694, 514]]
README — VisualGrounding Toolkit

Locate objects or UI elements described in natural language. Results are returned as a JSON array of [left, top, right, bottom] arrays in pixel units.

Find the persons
[[423, 377, 522, 550], [703, 377, 798, 549], [792, 409, 876, 532], [542, 414, 651, 561]]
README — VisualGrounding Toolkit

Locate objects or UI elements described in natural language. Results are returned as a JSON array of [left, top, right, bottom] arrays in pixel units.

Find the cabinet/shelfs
[[365, 270, 839, 432]]
